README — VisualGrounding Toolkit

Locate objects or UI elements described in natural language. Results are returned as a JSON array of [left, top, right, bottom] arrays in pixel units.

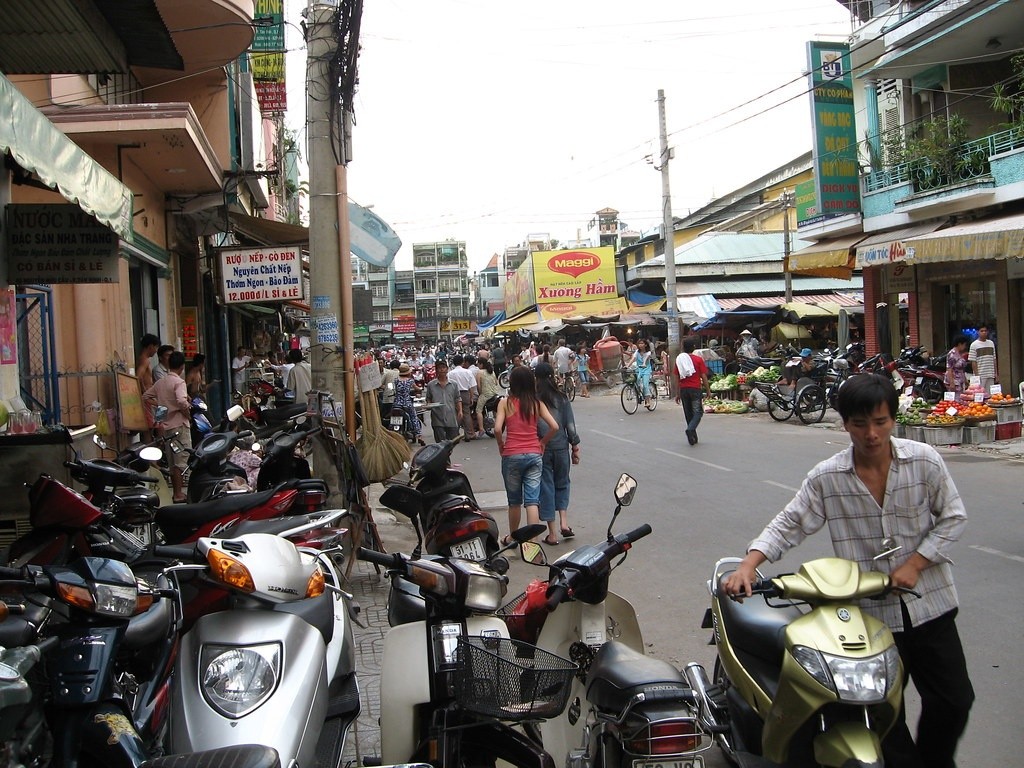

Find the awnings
[[782, 207, 1024, 281], [676, 295, 722, 318], [538, 297, 628, 320], [475, 297, 538, 332], [716, 294, 860, 310], [0, 71, 136, 245]]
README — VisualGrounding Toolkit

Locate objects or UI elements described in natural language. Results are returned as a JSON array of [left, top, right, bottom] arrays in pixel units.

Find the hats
[[741, 329, 752, 335], [535, 362, 554, 378], [399, 364, 412, 375], [799, 348, 812, 357], [435, 359, 447, 366]]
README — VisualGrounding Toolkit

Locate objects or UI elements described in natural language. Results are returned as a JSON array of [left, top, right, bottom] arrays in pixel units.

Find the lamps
[[985, 39, 1002, 49]]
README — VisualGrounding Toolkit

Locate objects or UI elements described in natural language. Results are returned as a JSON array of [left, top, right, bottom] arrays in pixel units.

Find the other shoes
[[777, 379, 787, 384], [418, 439, 426, 446], [685, 430, 698, 445], [789, 384, 795, 388], [476, 431, 486, 439], [644, 404, 650, 407], [462, 435, 471, 442], [172, 494, 187, 503], [470, 433, 476, 439]]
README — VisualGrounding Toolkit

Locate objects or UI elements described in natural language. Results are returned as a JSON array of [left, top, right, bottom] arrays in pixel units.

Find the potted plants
[[830, 51, 1024, 195]]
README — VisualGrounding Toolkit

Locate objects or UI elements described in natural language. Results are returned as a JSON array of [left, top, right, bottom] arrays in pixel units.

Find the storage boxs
[[890, 422, 965, 446], [963, 405, 1024, 445]]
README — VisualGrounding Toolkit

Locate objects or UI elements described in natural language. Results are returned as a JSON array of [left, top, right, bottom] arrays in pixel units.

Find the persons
[[138, 333, 222, 504], [964, 302, 994, 321], [674, 342, 711, 446], [735, 329, 762, 358], [623, 338, 653, 407], [626, 337, 667, 364], [905, 327, 910, 346], [721, 374, 977, 768], [747, 381, 777, 412], [371, 337, 591, 466], [709, 335, 720, 351], [533, 362, 580, 546], [944, 335, 970, 399], [772, 348, 816, 396], [233, 347, 252, 395], [493, 366, 560, 550], [266, 348, 312, 405], [971, 325, 997, 394]]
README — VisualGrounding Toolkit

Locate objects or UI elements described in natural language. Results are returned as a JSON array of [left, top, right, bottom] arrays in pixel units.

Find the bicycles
[[621, 365, 658, 415], [555, 375, 576, 403], [498, 368, 535, 388]]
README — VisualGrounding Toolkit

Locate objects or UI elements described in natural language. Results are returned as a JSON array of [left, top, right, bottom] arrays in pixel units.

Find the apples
[[960, 385, 988, 398], [932, 401, 965, 415]]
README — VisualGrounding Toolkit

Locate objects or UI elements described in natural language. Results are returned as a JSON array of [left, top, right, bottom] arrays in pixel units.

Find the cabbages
[[710, 380, 730, 391]]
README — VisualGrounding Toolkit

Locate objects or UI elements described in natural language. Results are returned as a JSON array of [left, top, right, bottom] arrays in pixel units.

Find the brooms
[[353, 350, 411, 482]]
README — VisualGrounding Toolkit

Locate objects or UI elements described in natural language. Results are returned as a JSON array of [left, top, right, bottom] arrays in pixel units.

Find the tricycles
[[755, 351, 863, 424]]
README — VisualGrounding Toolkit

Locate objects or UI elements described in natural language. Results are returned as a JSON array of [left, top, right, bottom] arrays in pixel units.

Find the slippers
[[500, 535, 518, 549], [561, 527, 575, 537], [542, 535, 559, 545]]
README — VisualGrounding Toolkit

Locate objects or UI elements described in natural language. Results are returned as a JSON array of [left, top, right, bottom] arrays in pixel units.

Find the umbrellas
[[836, 309, 849, 360]]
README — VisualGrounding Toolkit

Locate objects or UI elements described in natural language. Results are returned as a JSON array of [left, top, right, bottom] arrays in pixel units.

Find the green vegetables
[[745, 365, 781, 387], [708, 373, 737, 389], [701, 398, 748, 414]]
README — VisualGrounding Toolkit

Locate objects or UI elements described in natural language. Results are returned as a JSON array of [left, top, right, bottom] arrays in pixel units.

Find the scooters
[[409, 365, 423, 390], [424, 364, 436, 384], [0, 385, 923, 768], [736, 345, 949, 424]]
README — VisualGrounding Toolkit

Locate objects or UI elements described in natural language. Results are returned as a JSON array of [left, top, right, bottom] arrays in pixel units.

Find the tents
[[523, 302, 865, 348]]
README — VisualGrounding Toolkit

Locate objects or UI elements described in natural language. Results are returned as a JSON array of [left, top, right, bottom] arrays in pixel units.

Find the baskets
[[456, 634, 579, 719], [621, 371, 639, 383], [494, 580, 553, 643]]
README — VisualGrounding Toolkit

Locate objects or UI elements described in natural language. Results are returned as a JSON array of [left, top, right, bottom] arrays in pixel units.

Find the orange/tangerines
[[958, 402, 994, 416], [988, 394, 1013, 403]]
